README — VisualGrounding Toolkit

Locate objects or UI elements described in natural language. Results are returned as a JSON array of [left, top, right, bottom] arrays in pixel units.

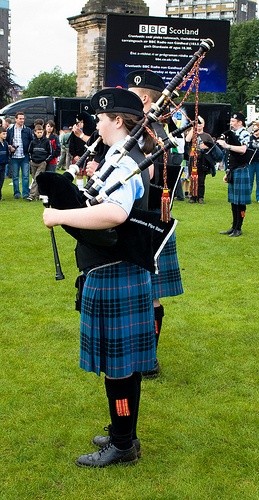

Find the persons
[[43, 87, 158, 468], [0, 70, 259, 377]]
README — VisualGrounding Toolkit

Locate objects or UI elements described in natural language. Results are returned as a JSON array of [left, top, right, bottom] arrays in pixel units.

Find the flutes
[[36, 37, 216, 280], [205, 117, 259, 171]]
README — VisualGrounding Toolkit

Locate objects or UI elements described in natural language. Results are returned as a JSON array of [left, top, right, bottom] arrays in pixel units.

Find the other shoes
[[219, 227, 234, 235], [199, 198, 204, 203], [15, 195, 21, 199], [27, 197, 33, 201], [229, 230, 242, 237], [188, 200, 195, 203]]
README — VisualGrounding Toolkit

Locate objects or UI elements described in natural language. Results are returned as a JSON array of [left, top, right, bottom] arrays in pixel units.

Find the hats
[[231, 112, 246, 121], [33, 124, 45, 131], [126, 70, 165, 92], [90, 88, 144, 117]]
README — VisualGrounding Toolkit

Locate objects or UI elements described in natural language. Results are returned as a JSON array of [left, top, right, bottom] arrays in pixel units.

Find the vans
[[0, 96, 93, 135]]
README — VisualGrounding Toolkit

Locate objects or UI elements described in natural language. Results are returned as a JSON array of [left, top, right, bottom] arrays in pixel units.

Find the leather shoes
[[92, 424, 140, 452], [75, 443, 139, 468]]
